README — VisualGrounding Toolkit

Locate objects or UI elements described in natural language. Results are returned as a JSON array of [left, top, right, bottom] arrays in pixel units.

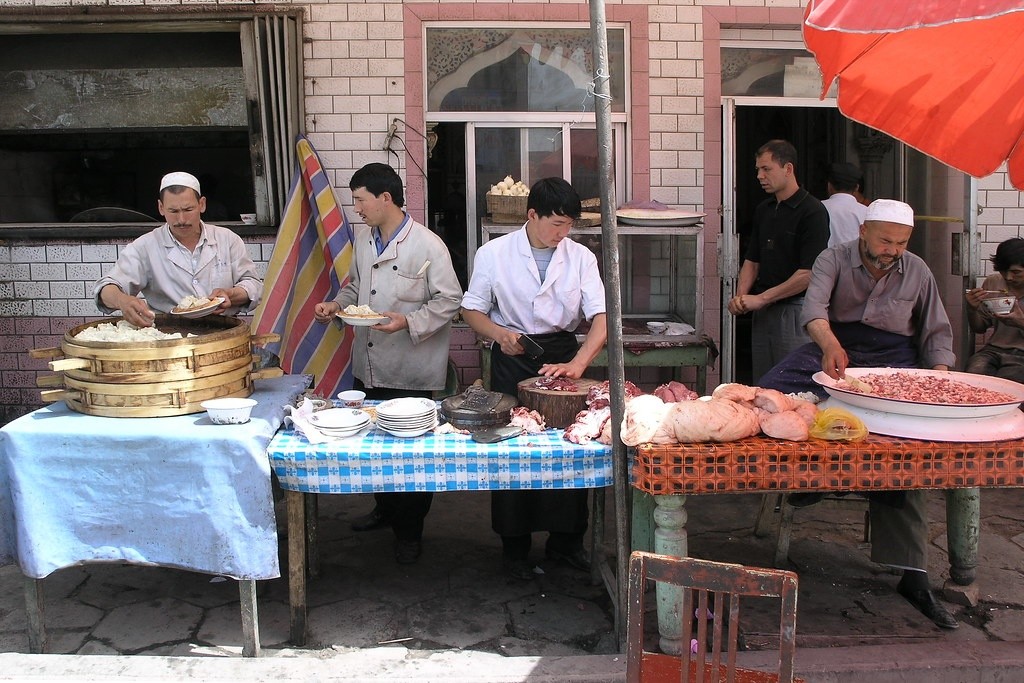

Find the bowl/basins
[[240, 214, 256, 225], [646, 322, 664, 334], [337, 390, 366, 409], [297, 400, 326, 412], [982, 296, 1016, 314], [200, 398, 258, 425]]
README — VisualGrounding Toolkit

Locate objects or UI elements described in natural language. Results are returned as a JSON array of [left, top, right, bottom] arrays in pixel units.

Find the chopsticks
[[416, 260, 431, 274], [965, 289, 1003, 293]]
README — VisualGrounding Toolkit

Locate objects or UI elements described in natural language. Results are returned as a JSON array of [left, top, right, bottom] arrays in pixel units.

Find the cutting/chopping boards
[[516, 376, 602, 428], [440, 391, 518, 432]]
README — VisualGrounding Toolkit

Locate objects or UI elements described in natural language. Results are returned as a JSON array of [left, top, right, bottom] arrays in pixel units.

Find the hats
[[865, 199, 915, 228], [159, 172, 201, 194]]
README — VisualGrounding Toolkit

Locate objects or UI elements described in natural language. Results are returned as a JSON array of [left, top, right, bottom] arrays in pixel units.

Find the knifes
[[458, 378, 504, 413], [516, 334, 544, 360]]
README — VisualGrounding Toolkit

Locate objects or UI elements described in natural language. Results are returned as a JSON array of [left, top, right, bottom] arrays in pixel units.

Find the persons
[[966, 238, 1024, 384], [313, 163, 464, 562], [823, 164, 868, 247], [857, 176, 871, 206], [756, 199, 960, 630], [95, 172, 264, 330], [727, 138, 830, 513], [462, 177, 607, 577]]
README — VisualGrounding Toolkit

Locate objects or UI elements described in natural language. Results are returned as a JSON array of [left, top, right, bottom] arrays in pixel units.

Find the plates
[[811, 367, 1024, 419], [616, 209, 708, 226], [375, 397, 439, 438], [307, 408, 371, 437], [816, 396, 1024, 442], [335, 313, 389, 326], [170, 298, 225, 319]]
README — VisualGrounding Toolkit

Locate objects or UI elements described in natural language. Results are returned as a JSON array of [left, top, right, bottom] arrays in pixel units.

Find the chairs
[[625, 551, 806, 683]]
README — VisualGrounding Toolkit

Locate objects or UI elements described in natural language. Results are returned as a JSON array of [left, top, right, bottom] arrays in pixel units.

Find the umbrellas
[[251, 132, 356, 399], [802, 0, 1024, 191]]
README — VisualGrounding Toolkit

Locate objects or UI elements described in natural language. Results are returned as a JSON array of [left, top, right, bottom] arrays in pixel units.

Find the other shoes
[[502, 553, 534, 579], [393, 536, 422, 563], [543, 545, 592, 572], [349, 510, 388, 531], [896, 578, 960, 628], [786, 490, 851, 508]]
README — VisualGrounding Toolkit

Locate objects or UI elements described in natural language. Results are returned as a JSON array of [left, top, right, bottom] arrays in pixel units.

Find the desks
[[272, 402, 626, 648], [627, 434, 1024, 653], [0, 373, 310, 659]]
